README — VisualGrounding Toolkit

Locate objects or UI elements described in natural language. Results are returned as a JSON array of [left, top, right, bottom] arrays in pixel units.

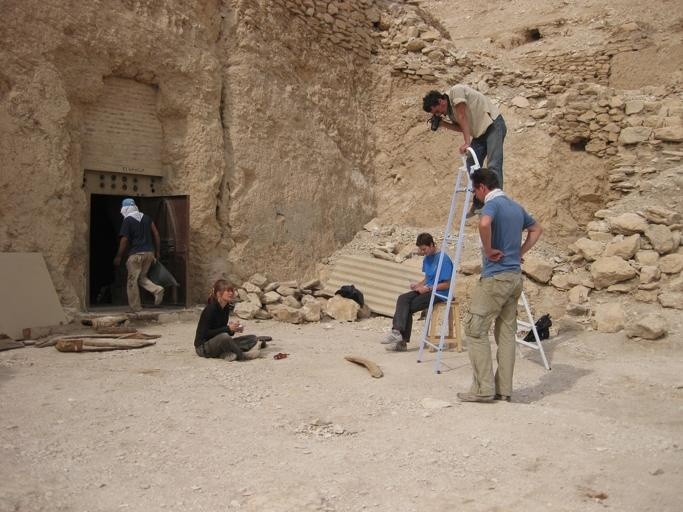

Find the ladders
[[417, 147, 552, 374]]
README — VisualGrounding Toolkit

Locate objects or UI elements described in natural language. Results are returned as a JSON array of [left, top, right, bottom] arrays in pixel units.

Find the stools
[[424, 300, 463, 353]]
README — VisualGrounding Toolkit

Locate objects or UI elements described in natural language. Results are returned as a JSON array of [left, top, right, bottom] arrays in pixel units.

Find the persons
[[422, 83, 507, 219], [380, 233, 453, 351], [194, 279, 261, 362], [456, 167, 543, 403], [113, 198, 165, 313]]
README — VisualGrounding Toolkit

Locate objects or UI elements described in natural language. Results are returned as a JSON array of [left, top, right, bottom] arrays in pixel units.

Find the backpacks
[[524, 312, 553, 342]]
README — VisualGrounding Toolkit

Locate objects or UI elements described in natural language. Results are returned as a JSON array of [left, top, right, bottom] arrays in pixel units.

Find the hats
[[122, 198, 136, 207]]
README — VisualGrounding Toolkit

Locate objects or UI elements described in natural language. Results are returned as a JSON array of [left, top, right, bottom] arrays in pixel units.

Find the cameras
[[429, 115, 441, 131]]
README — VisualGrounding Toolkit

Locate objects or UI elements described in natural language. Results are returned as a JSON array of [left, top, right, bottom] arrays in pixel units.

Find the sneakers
[[466, 204, 483, 219], [455, 390, 511, 403], [380, 329, 408, 352], [241, 350, 260, 361], [153, 287, 165, 306]]
